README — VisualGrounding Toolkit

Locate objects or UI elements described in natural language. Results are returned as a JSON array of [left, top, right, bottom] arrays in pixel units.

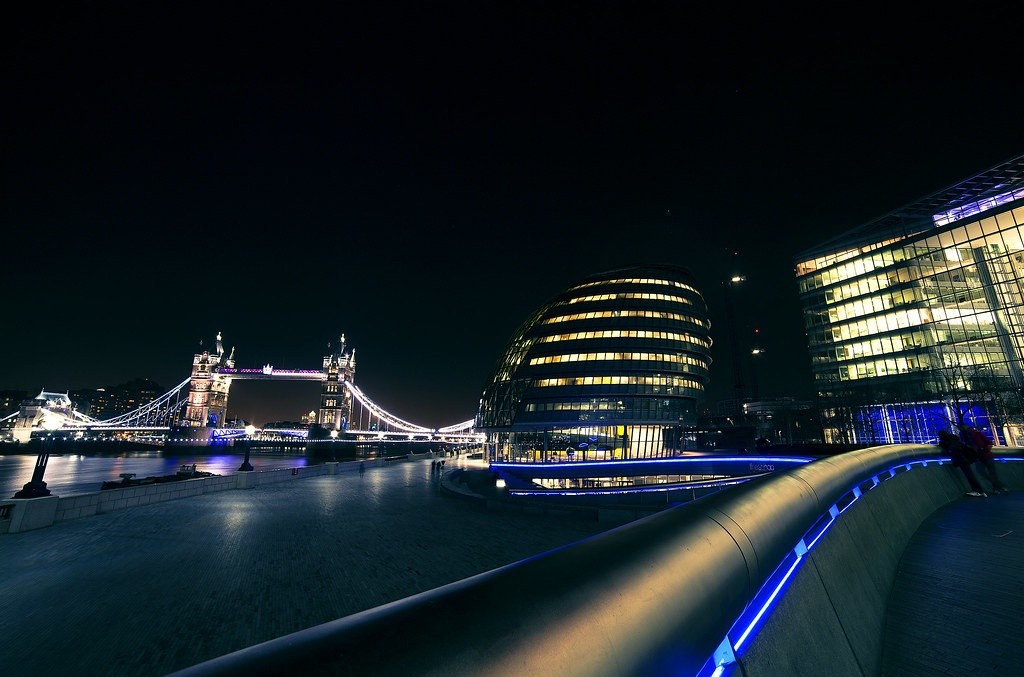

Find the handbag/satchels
[[965, 448, 978, 464]]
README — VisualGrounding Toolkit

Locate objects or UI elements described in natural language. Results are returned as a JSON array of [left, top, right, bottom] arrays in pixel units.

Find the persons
[[935, 420, 1011, 498]]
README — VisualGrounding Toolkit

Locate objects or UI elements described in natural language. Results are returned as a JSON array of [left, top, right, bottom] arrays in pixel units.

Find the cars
[[679, 433, 716, 452], [740, 437, 773, 456]]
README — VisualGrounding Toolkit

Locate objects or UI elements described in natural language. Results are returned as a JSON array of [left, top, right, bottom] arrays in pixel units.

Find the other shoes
[[966, 491, 988, 498], [993, 487, 1008, 495]]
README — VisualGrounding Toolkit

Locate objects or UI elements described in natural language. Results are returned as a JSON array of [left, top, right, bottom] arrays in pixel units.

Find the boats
[[164, 415, 248, 456], [100, 464, 220, 490]]
